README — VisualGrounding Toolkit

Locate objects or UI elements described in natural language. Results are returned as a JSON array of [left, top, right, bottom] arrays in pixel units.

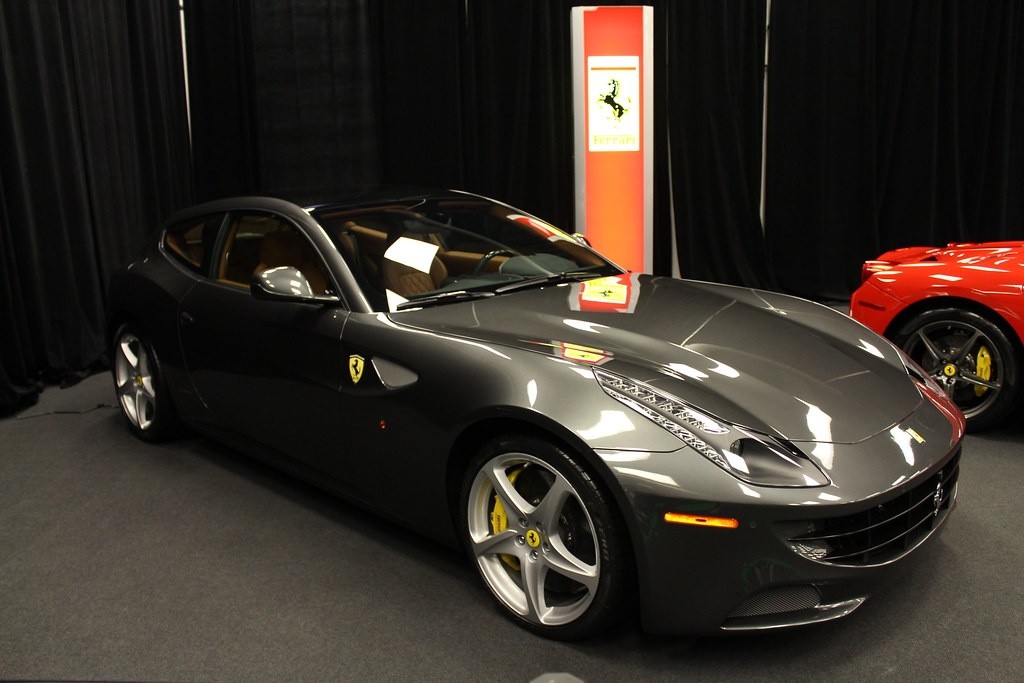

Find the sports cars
[[105, 181, 969, 644], [850, 238, 1024, 432]]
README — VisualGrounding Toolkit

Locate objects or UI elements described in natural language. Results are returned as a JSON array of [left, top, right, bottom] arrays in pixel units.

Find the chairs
[[380, 229, 447, 297], [253, 231, 328, 296]]
